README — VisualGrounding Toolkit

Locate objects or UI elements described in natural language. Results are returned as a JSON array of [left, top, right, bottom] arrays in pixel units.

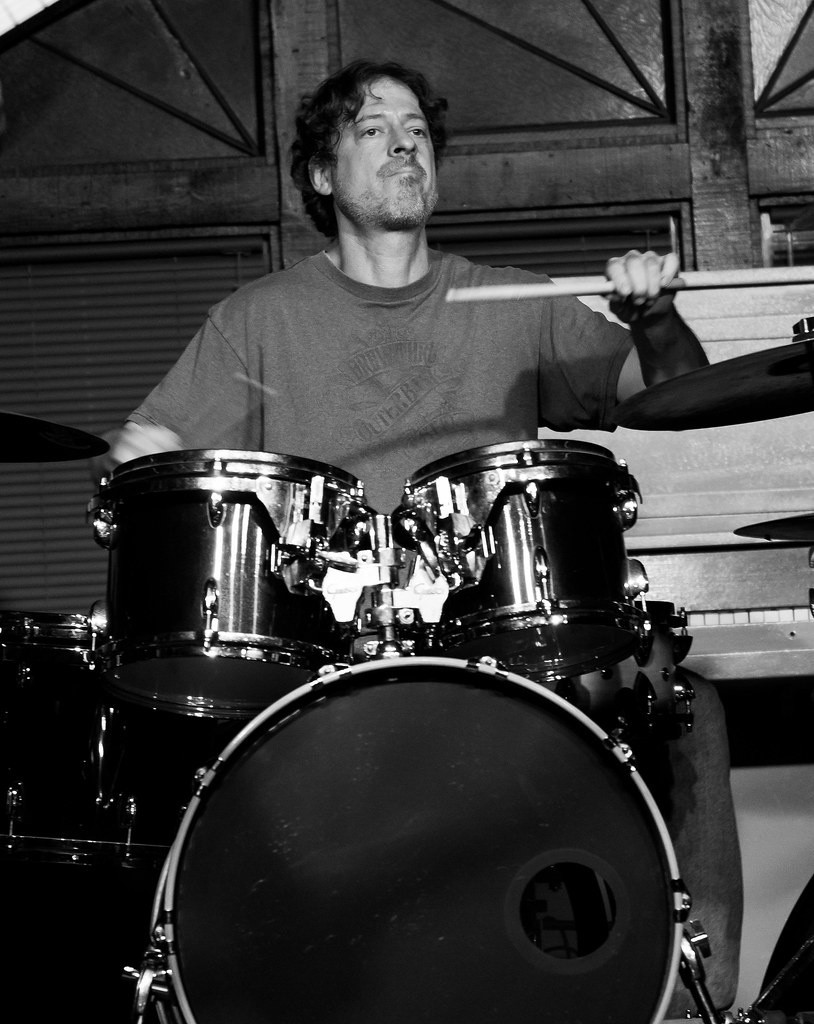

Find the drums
[[539, 601, 694, 740], [404, 437, 649, 685], [92, 449, 366, 722], [142, 652, 691, 1024], [0, 607, 138, 868]]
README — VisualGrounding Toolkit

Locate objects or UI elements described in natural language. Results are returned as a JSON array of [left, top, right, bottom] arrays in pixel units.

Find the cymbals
[[732, 513, 814, 541], [616, 339, 814, 432], [0, 411, 110, 463]]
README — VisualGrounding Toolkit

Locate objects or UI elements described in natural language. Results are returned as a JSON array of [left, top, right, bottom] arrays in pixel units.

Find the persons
[[87, 59, 744, 1021]]
